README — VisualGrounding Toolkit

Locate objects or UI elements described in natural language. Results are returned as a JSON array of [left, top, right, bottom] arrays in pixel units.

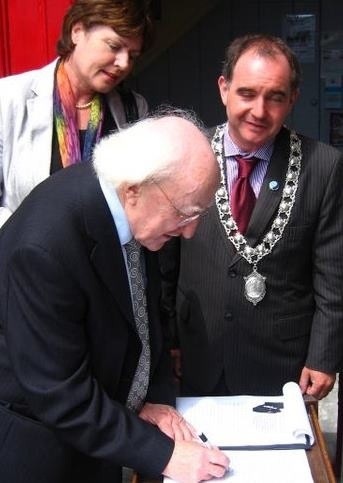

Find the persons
[[0, 117, 231, 483], [158, 35, 342, 401], [0, 0, 147, 232]]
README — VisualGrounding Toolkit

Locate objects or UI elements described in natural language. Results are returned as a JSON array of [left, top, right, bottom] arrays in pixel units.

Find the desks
[[160, 396, 336, 483]]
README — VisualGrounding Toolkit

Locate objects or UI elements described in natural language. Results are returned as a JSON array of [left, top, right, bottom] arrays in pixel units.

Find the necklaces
[[75, 100, 93, 109], [210, 119, 303, 303]]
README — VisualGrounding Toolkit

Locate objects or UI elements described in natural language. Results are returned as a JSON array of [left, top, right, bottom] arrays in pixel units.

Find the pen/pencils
[[198, 431, 213, 451]]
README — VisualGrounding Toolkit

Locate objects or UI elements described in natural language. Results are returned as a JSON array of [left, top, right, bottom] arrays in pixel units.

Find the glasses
[[152, 178, 209, 227]]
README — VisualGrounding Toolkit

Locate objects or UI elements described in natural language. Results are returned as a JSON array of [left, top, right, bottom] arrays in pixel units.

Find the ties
[[230, 154, 260, 236], [122, 238, 152, 483]]
